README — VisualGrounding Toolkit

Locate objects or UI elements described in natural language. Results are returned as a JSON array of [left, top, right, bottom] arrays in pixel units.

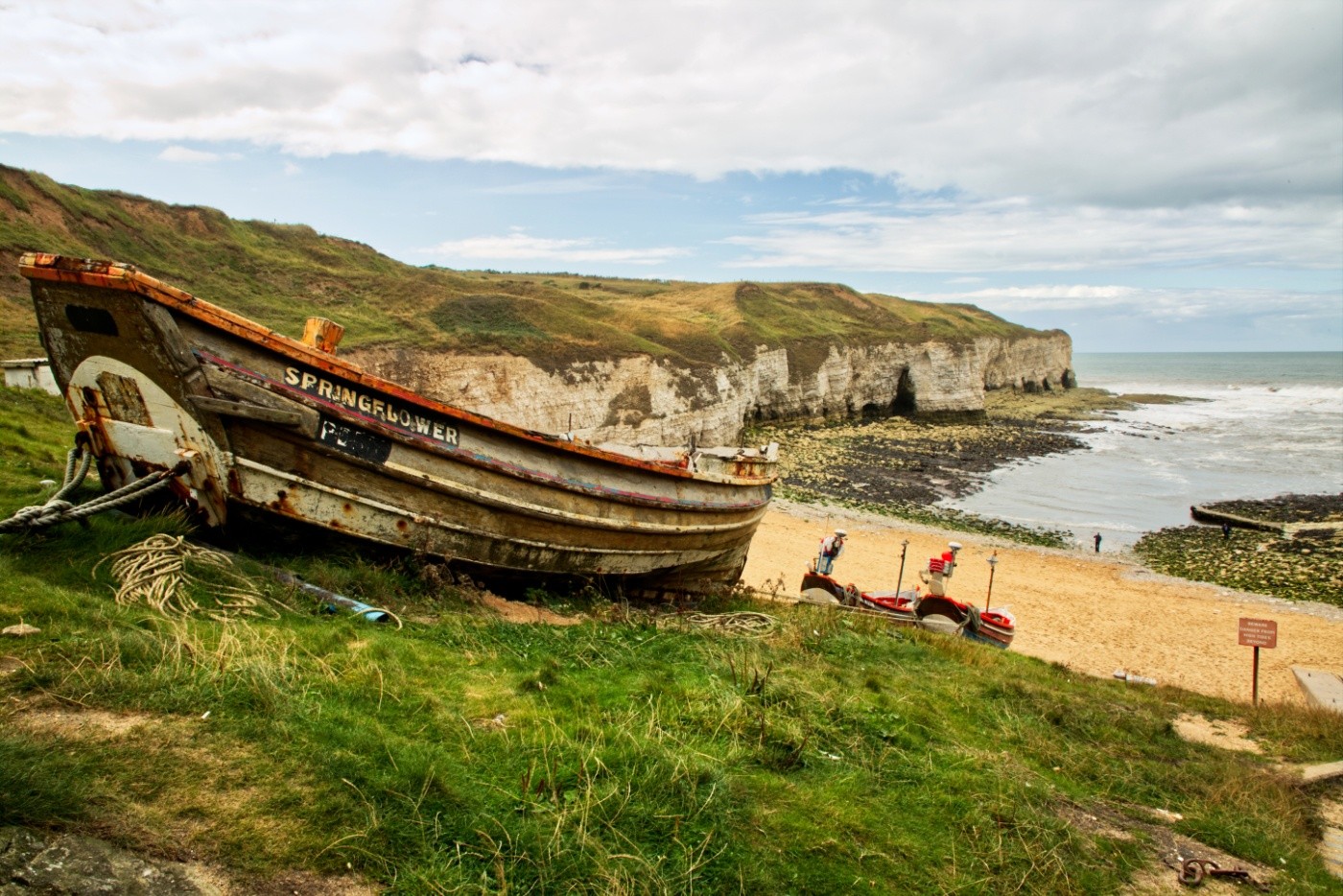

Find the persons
[[1221, 522, 1231, 539], [1094, 532, 1101, 553]]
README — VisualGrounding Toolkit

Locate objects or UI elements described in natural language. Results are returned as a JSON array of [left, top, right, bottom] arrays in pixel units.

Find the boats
[[18, 252, 780, 599], [913, 593, 1015, 650], [800, 557, 921, 625]]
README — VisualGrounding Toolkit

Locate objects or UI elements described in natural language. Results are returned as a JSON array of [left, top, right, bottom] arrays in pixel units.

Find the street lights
[[986, 549, 999, 614]]
